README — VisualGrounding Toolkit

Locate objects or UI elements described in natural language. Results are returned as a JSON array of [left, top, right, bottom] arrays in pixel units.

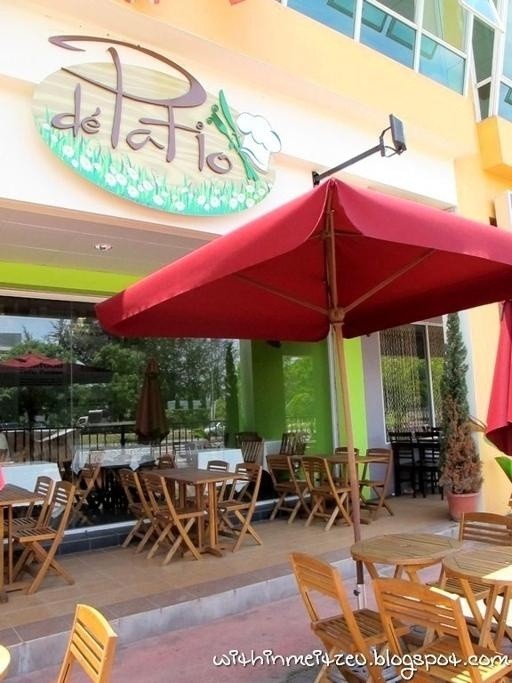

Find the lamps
[[310, 111, 406, 188]]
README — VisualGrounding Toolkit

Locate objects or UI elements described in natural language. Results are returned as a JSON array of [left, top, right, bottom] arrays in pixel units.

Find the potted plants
[[434, 393, 486, 523]]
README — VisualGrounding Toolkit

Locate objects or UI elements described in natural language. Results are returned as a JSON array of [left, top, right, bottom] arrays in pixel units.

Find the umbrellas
[[131, 359, 170, 457], [484, 299, 512, 457], [0, 350, 112, 462], [96, 179, 511, 608]]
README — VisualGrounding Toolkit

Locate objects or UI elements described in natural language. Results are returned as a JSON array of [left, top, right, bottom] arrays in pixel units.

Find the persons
[[0, 425, 10, 464]]
